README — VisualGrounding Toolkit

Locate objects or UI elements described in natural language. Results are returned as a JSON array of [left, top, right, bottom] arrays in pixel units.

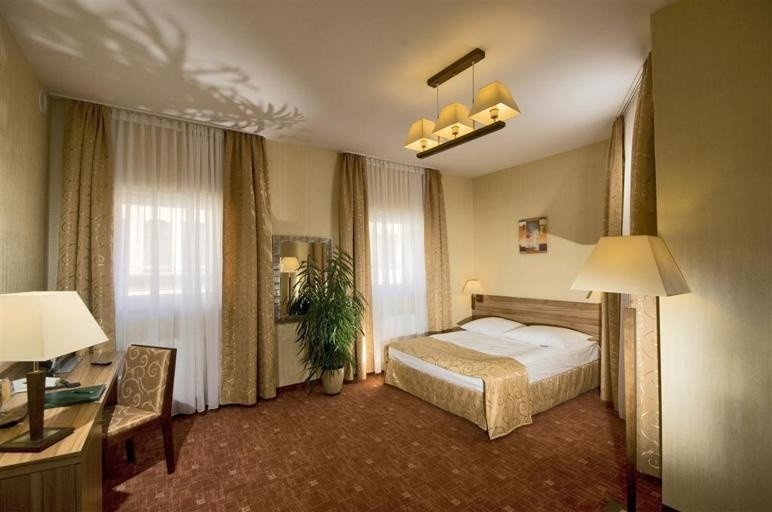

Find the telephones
[[12, 377, 61, 392]]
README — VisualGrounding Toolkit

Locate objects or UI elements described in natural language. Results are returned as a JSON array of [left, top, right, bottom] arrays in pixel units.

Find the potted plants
[[293, 244, 369, 398]]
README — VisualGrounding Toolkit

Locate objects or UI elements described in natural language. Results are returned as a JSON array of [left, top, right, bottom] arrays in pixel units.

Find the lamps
[[405, 48, 523, 160], [279, 256, 299, 316], [568, 234, 691, 511], [0, 290, 111, 451], [463, 280, 484, 309]]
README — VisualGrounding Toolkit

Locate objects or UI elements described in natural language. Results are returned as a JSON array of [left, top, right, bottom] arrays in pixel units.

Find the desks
[[1, 350, 125, 511]]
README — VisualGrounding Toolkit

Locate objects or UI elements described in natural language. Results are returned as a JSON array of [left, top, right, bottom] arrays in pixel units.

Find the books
[[11, 374, 61, 394]]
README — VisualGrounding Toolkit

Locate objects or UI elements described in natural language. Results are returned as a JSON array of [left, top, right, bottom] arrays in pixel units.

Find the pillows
[[461, 317, 592, 349]]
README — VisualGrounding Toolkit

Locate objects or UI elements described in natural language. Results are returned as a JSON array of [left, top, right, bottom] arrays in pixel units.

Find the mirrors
[[272, 235, 332, 324]]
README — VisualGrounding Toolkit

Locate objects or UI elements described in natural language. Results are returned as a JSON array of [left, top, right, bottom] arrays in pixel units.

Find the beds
[[383, 292, 601, 440]]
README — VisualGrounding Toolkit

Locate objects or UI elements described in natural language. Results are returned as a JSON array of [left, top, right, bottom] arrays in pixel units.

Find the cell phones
[[90, 360, 112, 366]]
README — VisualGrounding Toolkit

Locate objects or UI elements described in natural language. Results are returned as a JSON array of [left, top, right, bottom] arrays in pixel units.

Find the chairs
[[102, 344, 179, 479]]
